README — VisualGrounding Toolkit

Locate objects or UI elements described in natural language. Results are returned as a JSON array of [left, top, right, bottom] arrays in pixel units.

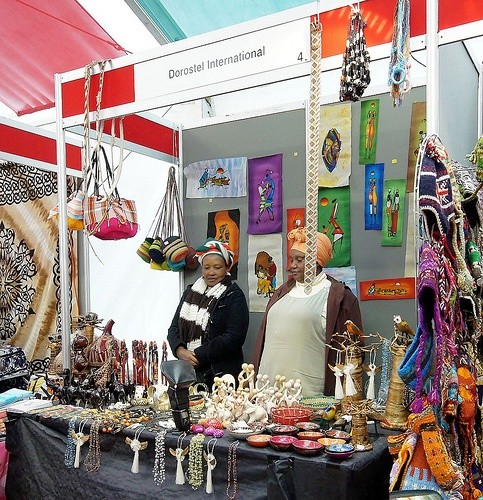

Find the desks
[[4, 399, 394, 500]]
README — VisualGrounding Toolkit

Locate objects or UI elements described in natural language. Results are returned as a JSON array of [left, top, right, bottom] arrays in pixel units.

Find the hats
[[194, 237, 235, 276]]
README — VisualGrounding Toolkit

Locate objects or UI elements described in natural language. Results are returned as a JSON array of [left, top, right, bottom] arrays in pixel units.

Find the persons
[[243, 227, 365, 398], [167, 236, 250, 395]]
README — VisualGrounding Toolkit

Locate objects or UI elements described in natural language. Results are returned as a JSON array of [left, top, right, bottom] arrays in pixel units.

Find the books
[[0, 388, 52, 442]]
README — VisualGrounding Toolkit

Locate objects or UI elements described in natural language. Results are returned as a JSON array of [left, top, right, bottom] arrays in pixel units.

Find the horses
[[46, 368, 134, 412]]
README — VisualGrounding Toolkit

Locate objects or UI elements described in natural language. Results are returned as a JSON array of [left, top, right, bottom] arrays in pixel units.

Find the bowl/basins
[[246, 421, 356, 459]]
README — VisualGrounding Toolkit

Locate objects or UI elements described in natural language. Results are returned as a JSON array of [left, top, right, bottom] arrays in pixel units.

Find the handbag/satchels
[[136, 236, 199, 273], [66, 190, 88, 231], [82, 197, 138, 240]]
